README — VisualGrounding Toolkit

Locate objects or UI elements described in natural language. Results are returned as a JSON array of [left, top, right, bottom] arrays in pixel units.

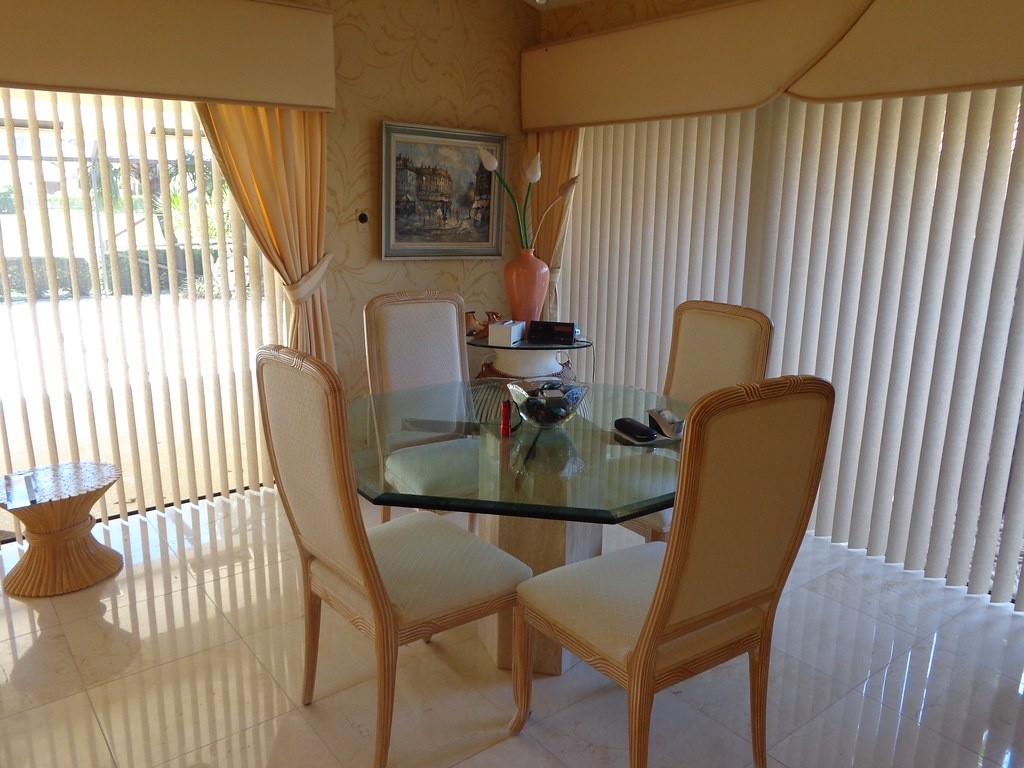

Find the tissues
[[488, 319, 526, 348]]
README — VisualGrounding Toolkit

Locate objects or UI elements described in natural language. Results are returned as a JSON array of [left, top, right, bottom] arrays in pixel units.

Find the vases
[[503, 246, 552, 331]]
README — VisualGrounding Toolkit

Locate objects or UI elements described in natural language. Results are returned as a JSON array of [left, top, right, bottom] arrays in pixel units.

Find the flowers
[[476, 146, 582, 248]]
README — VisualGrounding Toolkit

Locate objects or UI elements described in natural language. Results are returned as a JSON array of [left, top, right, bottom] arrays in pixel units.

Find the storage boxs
[[489, 319, 525, 347]]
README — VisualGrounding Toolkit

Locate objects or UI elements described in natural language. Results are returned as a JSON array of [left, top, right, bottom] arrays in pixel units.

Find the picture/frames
[[379, 120, 509, 261]]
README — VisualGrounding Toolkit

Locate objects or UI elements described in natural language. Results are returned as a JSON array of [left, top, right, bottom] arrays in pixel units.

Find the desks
[[466, 324, 594, 430], [344, 377, 695, 679], [0, 461, 124, 598]]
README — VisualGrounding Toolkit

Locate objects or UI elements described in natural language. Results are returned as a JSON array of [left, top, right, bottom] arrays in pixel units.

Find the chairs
[[361, 290, 482, 535], [505, 376, 836, 767], [257, 344, 535, 767], [602, 300, 774, 545]]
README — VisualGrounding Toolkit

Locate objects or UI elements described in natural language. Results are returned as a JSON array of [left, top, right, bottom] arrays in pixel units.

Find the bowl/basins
[[507, 376, 588, 428]]
